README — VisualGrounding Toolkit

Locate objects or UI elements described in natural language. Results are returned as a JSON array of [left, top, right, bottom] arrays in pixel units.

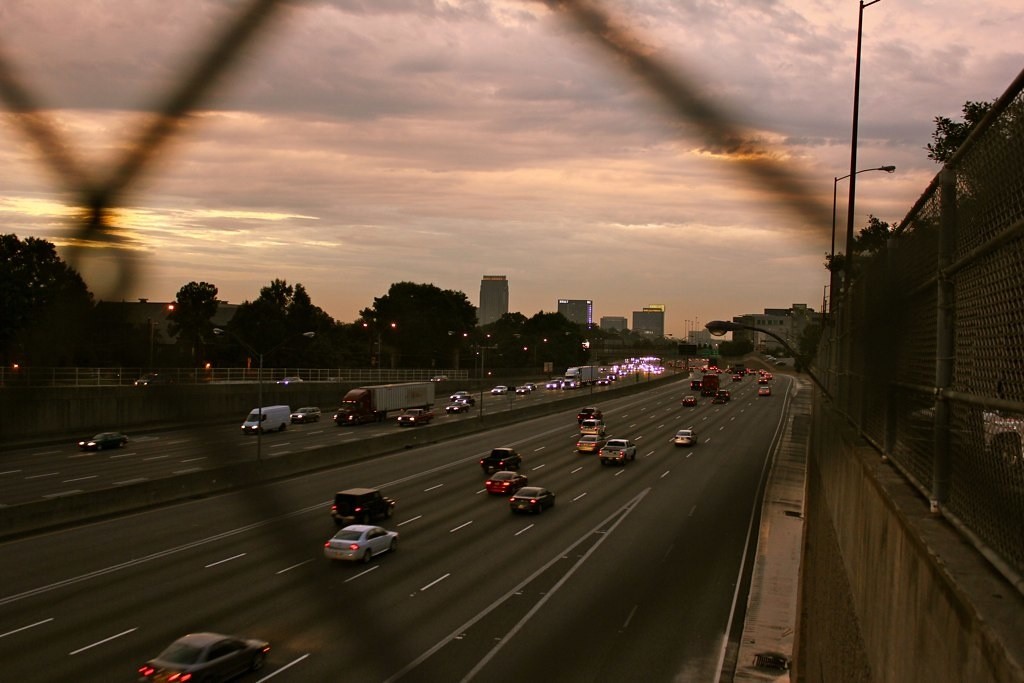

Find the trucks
[[564, 365, 600, 388]]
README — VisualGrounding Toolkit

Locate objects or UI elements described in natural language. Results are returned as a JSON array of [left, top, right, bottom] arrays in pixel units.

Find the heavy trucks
[[334, 381, 437, 426]]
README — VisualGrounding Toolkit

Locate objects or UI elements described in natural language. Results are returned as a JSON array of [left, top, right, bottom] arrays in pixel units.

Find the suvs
[[240, 405, 296, 434], [332, 487, 396, 526], [580, 418, 609, 437], [291, 406, 320, 423]]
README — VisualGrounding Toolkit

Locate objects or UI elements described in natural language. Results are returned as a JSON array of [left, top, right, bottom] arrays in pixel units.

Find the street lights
[[364, 322, 397, 371], [825, 163, 898, 392], [464, 334, 491, 379], [257, 331, 316, 459], [523, 338, 548, 368]]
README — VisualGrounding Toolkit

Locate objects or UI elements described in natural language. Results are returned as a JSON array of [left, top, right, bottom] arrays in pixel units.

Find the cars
[[78, 431, 137, 450], [444, 390, 476, 413], [674, 429, 700, 447], [431, 375, 449, 382], [596, 357, 667, 385], [140, 632, 270, 683], [545, 377, 565, 390], [685, 365, 773, 407], [486, 471, 534, 494], [491, 385, 509, 395], [578, 408, 605, 422], [509, 485, 558, 510], [515, 382, 538, 395], [323, 525, 398, 566], [576, 434, 604, 453]]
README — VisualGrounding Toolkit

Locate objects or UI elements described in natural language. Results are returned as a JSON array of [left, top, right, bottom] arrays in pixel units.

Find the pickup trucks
[[481, 448, 521, 473], [600, 438, 637, 464], [397, 408, 434, 425]]
